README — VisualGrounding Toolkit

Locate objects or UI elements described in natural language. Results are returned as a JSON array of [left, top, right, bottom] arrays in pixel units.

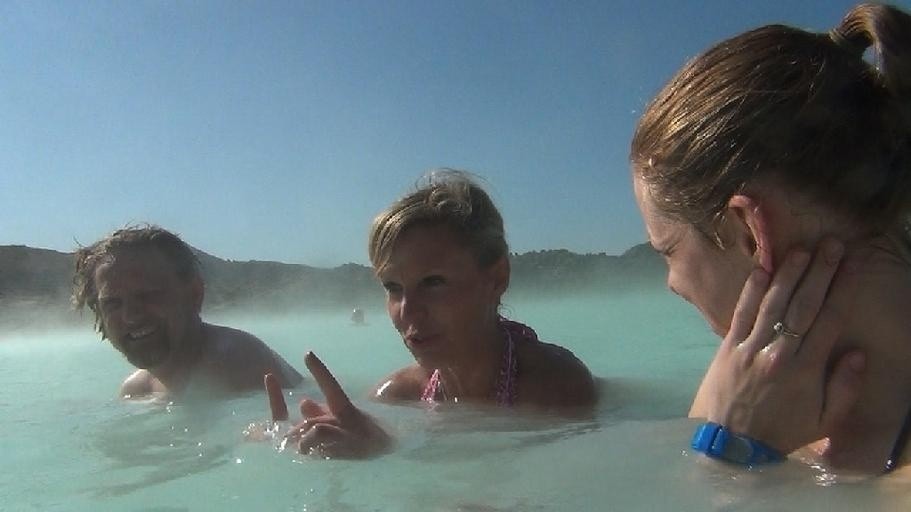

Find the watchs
[[690, 422, 792, 479]]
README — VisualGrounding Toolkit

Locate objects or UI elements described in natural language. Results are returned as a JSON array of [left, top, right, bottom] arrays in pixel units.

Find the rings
[[774, 322, 803, 340]]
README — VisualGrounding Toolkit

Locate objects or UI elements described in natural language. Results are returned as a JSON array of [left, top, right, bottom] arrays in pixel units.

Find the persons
[[628, 0, 910, 511], [348, 306, 371, 327], [59, 222, 303, 401], [241, 167, 598, 466]]
